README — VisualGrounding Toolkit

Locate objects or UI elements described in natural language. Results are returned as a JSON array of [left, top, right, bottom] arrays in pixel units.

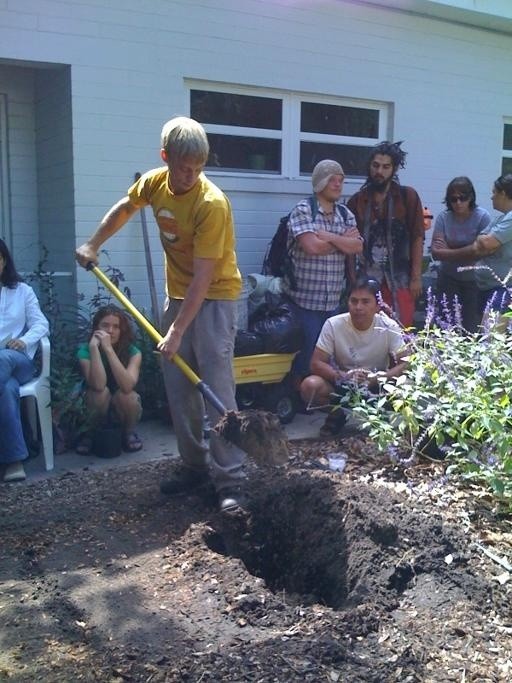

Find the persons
[[281, 159, 364, 414], [472, 173, 512, 347], [0, 236, 51, 480], [75, 302, 144, 455], [431, 176, 490, 340], [342, 139, 424, 333], [300, 276, 411, 437], [76, 115, 247, 513]]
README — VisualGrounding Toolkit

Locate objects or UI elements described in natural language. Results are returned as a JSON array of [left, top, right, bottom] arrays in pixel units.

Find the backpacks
[[260, 197, 348, 278]]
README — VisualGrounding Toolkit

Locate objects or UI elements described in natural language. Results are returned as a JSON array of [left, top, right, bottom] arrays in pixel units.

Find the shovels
[[87, 263, 290, 465]]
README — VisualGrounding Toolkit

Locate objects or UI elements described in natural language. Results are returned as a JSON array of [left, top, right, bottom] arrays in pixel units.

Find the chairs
[[12, 336, 55, 473]]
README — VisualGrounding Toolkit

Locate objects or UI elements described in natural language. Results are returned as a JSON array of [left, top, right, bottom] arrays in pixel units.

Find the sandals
[[121, 431, 143, 453], [75, 434, 95, 456]]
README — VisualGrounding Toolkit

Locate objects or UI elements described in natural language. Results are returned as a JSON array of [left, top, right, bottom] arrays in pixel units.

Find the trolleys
[[218, 342, 302, 426]]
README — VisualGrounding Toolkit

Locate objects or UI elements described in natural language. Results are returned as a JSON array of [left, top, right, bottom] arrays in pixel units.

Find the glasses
[[447, 194, 469, 203]]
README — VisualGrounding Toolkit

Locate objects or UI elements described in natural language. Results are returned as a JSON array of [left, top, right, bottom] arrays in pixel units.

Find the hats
[[311, 159, 345, 193]]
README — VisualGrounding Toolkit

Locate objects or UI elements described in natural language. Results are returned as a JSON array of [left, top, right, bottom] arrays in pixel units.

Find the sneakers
[[319, 414, 349, 437], [160, 465, 212, 498], [205, 484, 240, 515], [3, 464, 26, 482]]
